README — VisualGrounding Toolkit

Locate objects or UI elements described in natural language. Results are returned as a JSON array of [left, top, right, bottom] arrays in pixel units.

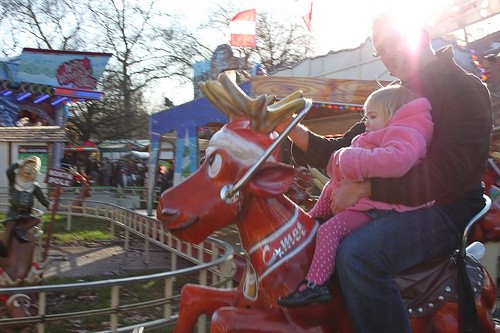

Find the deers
[[156, 72, 500, 333]]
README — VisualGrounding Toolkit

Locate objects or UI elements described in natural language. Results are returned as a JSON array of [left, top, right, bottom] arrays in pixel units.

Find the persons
[[276, 20, 494, 333], [75, 156, 174, 207], [275, 83, 437, 307], [0, 154, 62, 256]]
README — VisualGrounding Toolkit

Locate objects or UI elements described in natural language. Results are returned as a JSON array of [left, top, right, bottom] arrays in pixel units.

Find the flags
[[302, 2, 313, 31], [229, 8, 257, 47]]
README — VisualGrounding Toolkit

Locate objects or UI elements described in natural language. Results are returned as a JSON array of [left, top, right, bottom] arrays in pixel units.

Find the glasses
[[371, 36, 421, 58]]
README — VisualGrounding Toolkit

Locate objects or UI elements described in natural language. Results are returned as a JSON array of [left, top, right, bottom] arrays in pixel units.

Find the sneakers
[[277, 279, 334, 306]]
